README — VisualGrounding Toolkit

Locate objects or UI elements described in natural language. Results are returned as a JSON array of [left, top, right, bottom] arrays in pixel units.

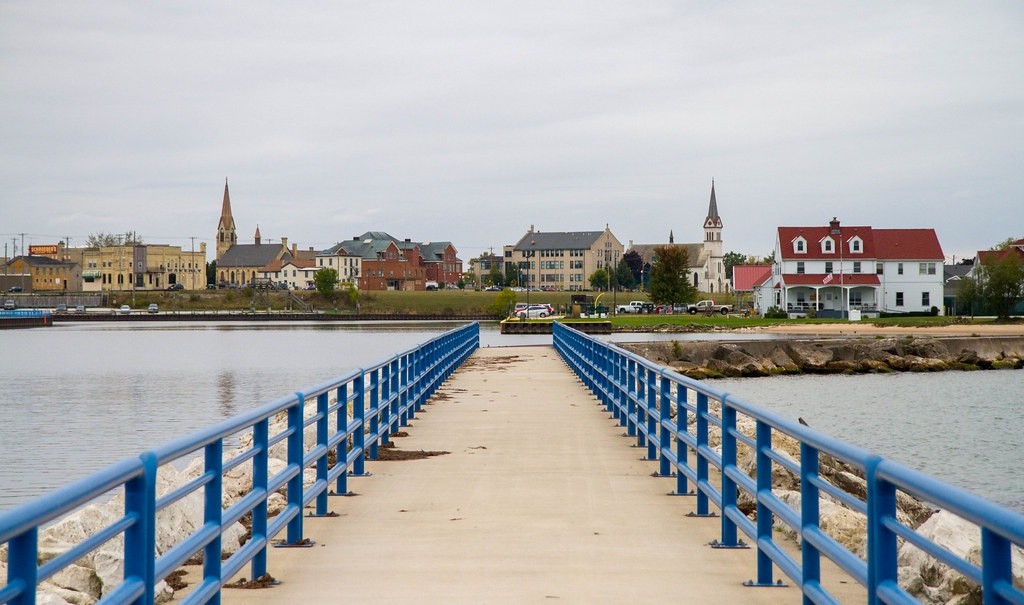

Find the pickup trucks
[[617, 301, 647, 314], [687, 300, 734, 315]]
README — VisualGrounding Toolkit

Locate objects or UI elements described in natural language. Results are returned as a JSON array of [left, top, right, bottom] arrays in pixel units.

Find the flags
[[823, 273, 833, 284]]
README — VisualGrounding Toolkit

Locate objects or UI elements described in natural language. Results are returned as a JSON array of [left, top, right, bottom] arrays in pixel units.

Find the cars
[[56, 304, 69, 313], [7, 286, 26, 293], [484, 285, 500, 291], [75, 305, 87, 314], [120, 304, 132, 315], [511, 286, 557, 291], [206, 283, 317, 290], [168, 284, 184, 290], [514, 302, 555, 317], [4, 299, 16, 310], [148, 303, 159, 313]]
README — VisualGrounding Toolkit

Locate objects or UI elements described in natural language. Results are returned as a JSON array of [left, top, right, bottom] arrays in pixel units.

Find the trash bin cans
[[520, 312, 525, 322], [573, 304, 580, 317]]
